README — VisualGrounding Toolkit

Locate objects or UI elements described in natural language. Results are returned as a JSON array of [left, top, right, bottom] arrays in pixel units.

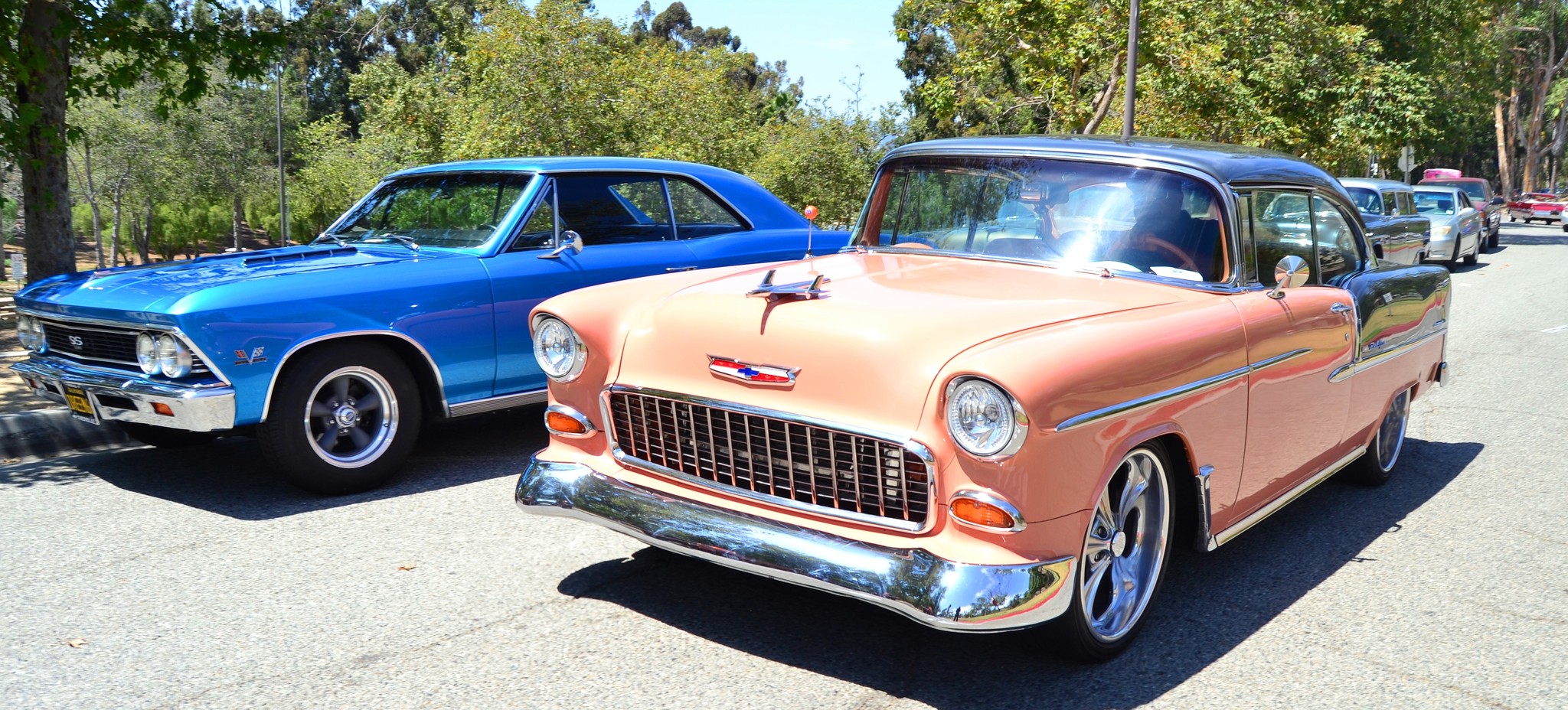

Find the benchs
[[563, 221, 742, 247], [1252, 240, 1356, 287]]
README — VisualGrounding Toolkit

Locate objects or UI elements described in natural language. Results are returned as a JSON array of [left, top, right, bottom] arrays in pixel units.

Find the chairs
[[1435, 194, 1453, 210]]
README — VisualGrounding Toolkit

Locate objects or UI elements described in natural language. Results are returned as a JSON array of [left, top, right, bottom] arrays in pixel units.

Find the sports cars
[[511, 135, 1459, 666]]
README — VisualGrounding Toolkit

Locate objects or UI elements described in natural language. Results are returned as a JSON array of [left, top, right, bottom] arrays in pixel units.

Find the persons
[[1092, 168, 1222, 282], [1437, 199, 1452, 211]]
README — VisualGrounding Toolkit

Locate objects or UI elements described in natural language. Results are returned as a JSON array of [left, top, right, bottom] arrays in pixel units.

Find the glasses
[[1130, 191, 1182, 202]]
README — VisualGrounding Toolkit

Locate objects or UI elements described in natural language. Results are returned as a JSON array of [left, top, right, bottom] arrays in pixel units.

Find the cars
[[14, 156, 948, 498], [1507, 192, 1568, 226], [1561, 202, 1568, 232], [1537, 184, 1568, 194], [1491, 194, 1506, 205], [935, 173, 1505, 274]]
